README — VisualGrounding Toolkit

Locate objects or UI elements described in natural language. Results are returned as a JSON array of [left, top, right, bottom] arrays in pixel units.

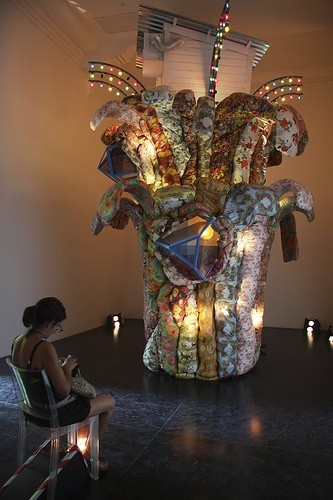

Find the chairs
[[5, 357, 99, 500]]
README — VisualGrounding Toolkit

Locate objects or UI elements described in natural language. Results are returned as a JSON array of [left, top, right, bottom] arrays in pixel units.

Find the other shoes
[[86, 459, 109, 469]]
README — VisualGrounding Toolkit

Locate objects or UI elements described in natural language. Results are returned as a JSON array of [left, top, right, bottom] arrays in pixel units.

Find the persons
[[10, 296, 116, 471]]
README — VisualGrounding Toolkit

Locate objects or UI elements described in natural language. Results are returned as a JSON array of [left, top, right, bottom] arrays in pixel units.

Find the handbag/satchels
[[60, 356, 96, 399]]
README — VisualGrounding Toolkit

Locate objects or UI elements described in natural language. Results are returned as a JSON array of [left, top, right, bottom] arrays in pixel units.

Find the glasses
[[55, 323, 63, 332]]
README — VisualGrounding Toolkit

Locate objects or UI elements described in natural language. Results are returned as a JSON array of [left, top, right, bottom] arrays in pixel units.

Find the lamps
[[303, 318, 320, 337], [106, 312, 124, 328]]
